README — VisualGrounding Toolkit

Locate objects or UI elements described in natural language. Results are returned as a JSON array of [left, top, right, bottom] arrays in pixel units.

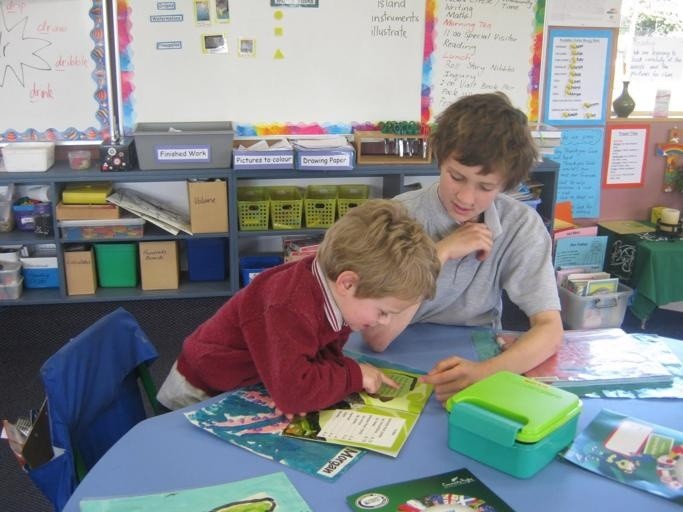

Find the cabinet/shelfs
[[0, 156, 559, 309]]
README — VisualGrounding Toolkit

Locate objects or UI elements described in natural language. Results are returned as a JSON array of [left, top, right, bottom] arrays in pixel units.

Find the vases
[[613, 79, 635, 116]]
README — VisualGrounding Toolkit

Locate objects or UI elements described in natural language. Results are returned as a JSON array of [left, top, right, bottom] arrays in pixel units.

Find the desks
[[59, 323, 680, 511], [596, 220, 683, 329]]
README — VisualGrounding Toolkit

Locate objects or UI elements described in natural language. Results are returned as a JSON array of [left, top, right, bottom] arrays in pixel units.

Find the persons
[[360, 92, 563, 408], [155, 198, 440, 414]]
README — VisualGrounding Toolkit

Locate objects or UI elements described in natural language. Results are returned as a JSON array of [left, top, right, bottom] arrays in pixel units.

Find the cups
[[653, 88, 671, 119]]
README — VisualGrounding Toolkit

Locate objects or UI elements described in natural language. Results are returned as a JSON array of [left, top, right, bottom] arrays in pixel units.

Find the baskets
[[236, 183, 370, 230]]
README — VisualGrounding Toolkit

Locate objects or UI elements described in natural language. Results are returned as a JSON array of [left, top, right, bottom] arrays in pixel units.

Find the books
[[556, 265, 619, 296], [495, 328, 672, 388], [282, 236, 320, 262], [527, 123, 561, 155]]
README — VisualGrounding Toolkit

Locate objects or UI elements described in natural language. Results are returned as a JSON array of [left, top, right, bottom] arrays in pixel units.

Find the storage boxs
[[240, 253, 284, 287], [0, 182, 147, 237], [0, 142, 55, 174], [1, 239, 225, 302], [187, 176, 228, 235], [131, 119, 357, 171], [553, 269, 633, 331]]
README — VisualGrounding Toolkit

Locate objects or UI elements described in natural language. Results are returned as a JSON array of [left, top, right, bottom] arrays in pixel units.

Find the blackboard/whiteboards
[[0, 0, 117, 147], [111, 1, 546, 145]]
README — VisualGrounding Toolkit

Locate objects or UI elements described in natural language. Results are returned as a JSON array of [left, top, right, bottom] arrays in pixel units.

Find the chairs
[[39, 307, 164, 486]]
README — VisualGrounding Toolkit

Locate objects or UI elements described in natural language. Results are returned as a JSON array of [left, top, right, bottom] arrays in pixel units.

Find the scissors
[[382, 121, 431, 159]]
